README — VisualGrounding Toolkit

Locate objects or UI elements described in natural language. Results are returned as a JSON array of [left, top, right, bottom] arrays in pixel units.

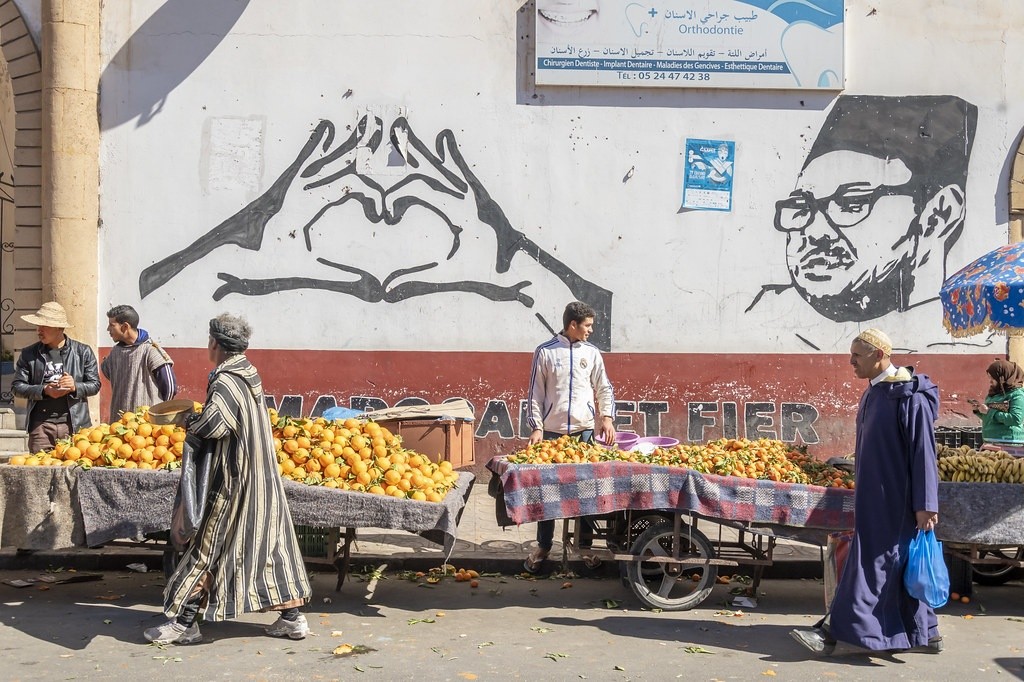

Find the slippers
[[579, 549, 601, 570], [523, 551, 550, 572]]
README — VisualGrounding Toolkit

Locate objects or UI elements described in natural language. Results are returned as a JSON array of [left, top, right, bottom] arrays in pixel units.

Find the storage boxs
[[374, 417, 476, 469], [932, 426, 984, 451], [294, 524, 340, 557]]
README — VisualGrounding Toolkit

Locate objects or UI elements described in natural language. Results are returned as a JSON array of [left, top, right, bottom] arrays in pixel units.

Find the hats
[[857, 329, 892, 357], [20, 301, 73, 328]]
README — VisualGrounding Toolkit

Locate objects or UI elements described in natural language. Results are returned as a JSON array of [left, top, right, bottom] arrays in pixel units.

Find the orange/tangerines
[[508, 434, 855, 491], [563, 574, 732, 588], [415, 565, 481, 588], [951, 593, 969, 604], [9, 401, 460, 502]]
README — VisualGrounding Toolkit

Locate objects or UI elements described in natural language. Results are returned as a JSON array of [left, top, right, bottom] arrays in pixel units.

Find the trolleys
[[132, 519, 359, 593], [560, 507, 779, 612], [935, 540, 1024, 587]]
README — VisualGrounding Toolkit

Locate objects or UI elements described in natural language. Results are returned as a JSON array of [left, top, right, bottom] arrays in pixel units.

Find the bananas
[[937, 442, 1024, 483]]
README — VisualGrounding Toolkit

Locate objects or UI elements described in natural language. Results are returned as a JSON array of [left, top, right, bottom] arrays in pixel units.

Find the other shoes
[[789, 628, 835, 656], [891, 637, 942, 654]]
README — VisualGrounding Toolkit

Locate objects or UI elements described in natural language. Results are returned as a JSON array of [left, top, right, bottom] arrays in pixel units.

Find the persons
[[971, 360, 1024, 448], [524, 302, 616, 572], [144, 311, 312, 645], [101, 305, 176, 425], [10, 302, 103, 453], [792, 327, 943, 657]]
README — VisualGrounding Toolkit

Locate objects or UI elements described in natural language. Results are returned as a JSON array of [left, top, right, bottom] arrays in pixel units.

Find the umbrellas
[[939, 240, 1024, 339]]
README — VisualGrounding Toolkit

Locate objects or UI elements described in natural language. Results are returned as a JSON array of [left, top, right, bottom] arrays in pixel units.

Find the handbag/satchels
[[904, 528, 951, 608]]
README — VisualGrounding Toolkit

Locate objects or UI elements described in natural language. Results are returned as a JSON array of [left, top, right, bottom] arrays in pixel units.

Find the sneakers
[[144, 618, 202, 644], [265, 612, 308, 639]]
